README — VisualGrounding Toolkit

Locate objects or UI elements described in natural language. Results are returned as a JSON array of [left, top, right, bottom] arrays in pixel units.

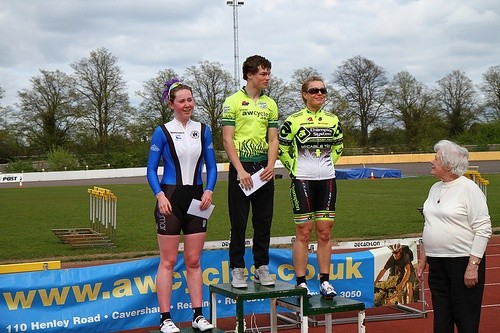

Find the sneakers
[[254, 265, 275, 285], [298, 283, 313, 298], [192, 315, 215, 331], [159, 318, 180, 333], [231, 267, 248, 287], [320, 280, 337, 298]]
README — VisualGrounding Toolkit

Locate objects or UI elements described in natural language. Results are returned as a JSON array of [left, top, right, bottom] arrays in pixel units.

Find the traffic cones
[[370, 171, 375, 180], [17, 180, 24, 188]]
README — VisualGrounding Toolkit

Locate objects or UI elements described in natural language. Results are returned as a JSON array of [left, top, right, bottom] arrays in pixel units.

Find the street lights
[[227, 0, 244, 93]]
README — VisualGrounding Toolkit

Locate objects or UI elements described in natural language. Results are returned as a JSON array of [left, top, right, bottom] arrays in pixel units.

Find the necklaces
[[437, 180, 455, 204]]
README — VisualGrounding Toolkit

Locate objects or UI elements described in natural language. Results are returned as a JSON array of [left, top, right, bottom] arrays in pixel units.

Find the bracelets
[[468, 261, 480, 266]]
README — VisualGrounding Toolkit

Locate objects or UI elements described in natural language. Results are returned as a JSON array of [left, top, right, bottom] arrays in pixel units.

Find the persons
[[374, 244, 415, 306], [223, 55, 279, 288], [146, 79, 217, 333], [416, 140, 492, 333], [278, 76, 344, 300]]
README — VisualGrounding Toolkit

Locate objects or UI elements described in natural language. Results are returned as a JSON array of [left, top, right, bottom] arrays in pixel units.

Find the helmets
[[393, 243, 402, 251]]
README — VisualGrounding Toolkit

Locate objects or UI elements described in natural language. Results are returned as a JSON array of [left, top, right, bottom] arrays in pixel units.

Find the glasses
[[306, 87, 327, 94], [392, 250, 400, 254], [169, 81, 191, 99]]
